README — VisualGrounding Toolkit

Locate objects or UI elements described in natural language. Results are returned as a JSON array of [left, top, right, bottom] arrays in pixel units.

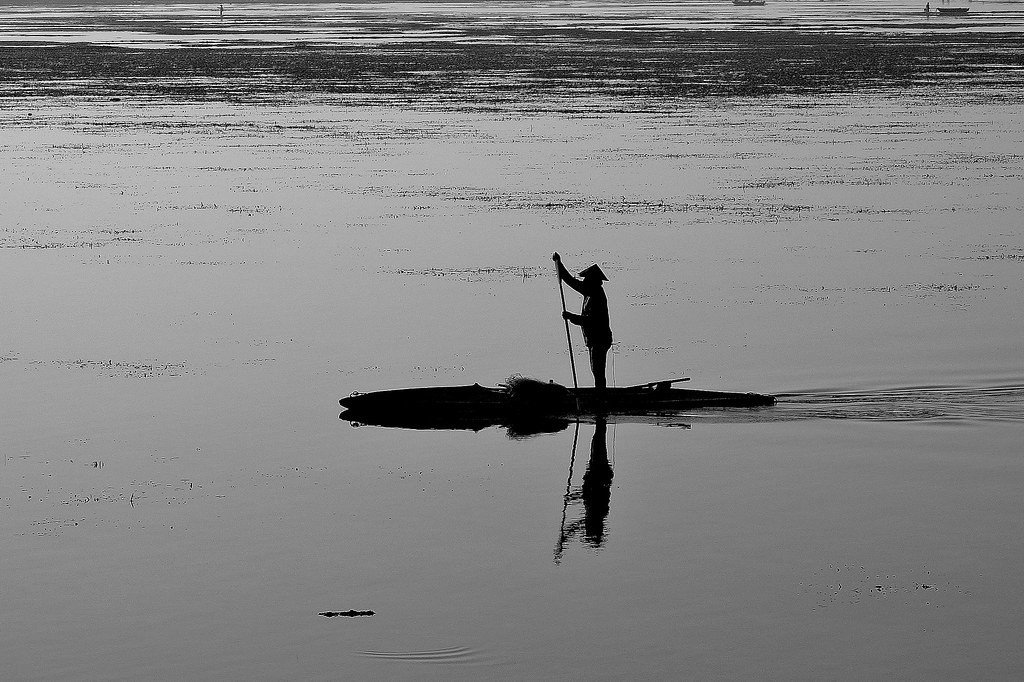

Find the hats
[[579, 264, 609, 281]]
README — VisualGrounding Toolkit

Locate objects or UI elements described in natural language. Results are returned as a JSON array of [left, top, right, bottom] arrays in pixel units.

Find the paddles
[[625, 377, 690, 390], [553, 252, 578, 387]]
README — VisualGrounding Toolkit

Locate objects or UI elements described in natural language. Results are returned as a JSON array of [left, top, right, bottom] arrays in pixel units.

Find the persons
[[553, 253, 612, 391], [556, 420, 615, 551]]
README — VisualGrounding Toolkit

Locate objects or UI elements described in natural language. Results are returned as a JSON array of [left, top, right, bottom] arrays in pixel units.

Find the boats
[[337, 374, 778, 415]]
